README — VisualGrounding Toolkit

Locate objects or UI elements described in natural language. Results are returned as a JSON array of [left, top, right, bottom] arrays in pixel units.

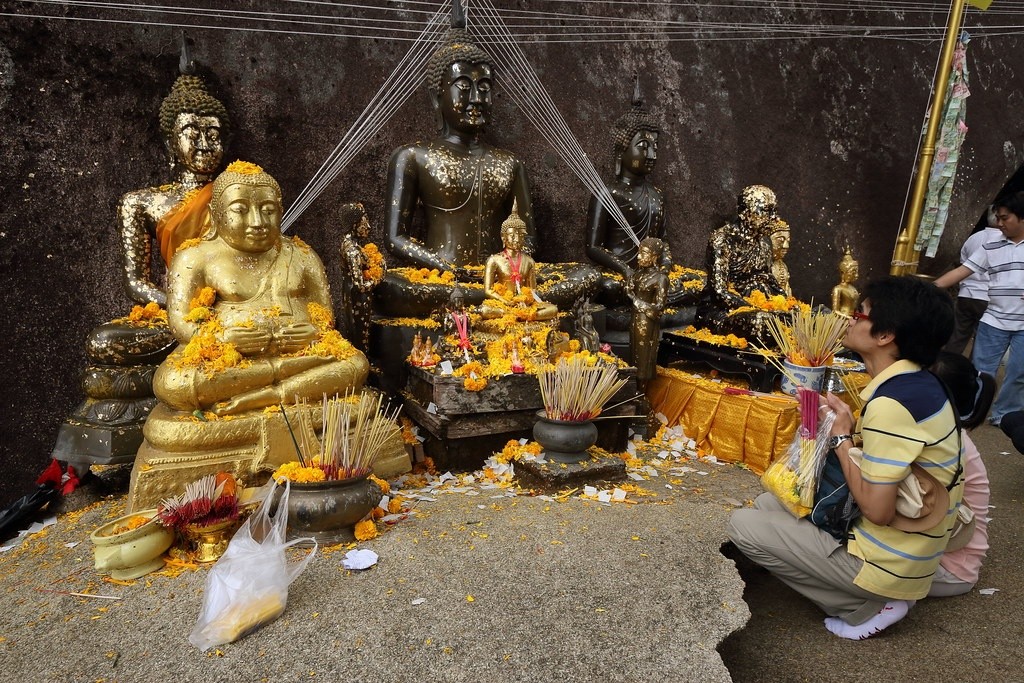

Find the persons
[[583, 105, 707, 305], [932, 187, 1024, 427], [479, 196, 559, 320], [86, 74, 227, 363], [440, 279, 486, 351], [338, 203, 387, 352], [152, 171, 370, 416], [379, 27, 602, 305], [725, 275, 965, 640], [943, 205, 1002, 361], [832, 251, 860, 343], [622, 238, 670, 392], [929, 350, 990, 596], [693, 185, 816, 347], [769, 219, 792, 297]]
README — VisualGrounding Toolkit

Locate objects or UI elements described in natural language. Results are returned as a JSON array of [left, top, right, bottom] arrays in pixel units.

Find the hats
[[847, 446, 950, 532]]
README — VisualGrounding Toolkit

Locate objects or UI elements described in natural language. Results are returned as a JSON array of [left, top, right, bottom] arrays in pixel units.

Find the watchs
[[829, 434, 852, 449]]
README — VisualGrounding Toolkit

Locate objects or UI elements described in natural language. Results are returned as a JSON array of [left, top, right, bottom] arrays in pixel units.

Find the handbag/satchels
[[188, 476, 317, 652], [812, 449, 862, 544], [759, 404, 837, 519]]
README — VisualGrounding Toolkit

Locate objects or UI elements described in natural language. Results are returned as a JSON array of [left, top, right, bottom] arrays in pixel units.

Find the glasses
[[853, 308, 873, 322]]
